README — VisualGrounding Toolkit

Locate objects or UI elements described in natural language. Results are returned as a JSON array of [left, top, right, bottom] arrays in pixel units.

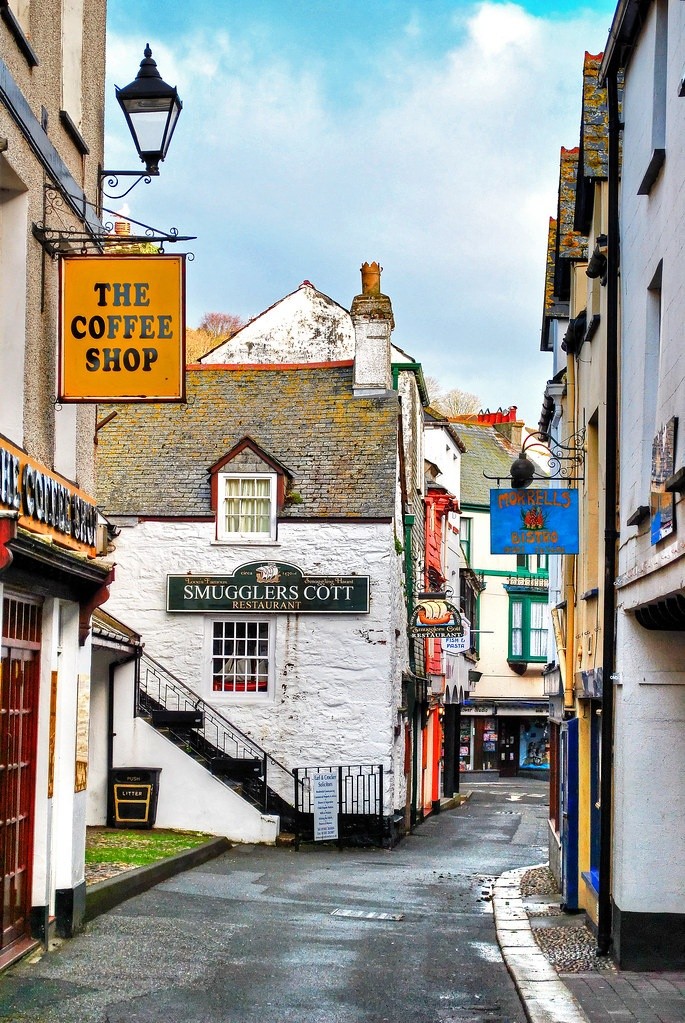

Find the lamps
[[98, 44, 185, 217], [509, 408, 587, 486]]
